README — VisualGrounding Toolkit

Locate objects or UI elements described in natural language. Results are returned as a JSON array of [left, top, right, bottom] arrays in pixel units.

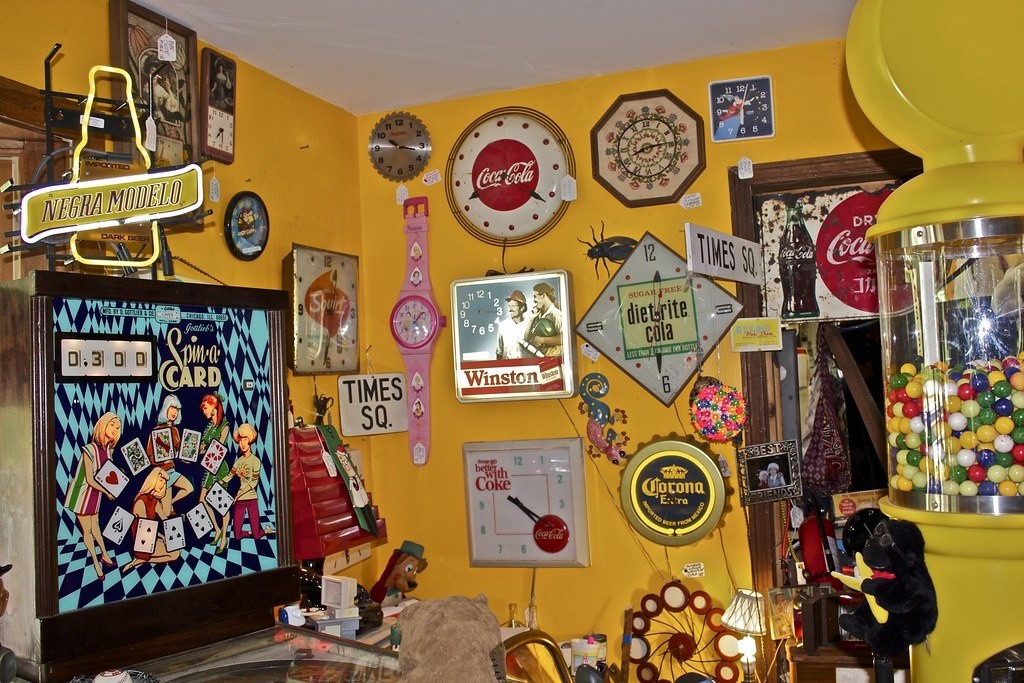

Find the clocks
[[615, 433, 733, 547], [590, 88, 707, 208], [367, 111, 432, 184], [444, 106, 577, 247], [281, 242, 360, 377], [572, 230, 745, 410], [461, 436, 591, 569], [200, 48, 237, 166], [108, 0, 205, 231], [448, 270, 575, 404], [708, 75, 775, 143]]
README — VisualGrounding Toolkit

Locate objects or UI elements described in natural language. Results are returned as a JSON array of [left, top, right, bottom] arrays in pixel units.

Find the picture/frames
[[735, 438, 802, 506], [224, 190, 270, 262]]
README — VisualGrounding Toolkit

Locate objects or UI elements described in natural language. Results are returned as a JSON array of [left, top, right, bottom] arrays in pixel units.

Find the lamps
[[720, 587, 767, 682]]
